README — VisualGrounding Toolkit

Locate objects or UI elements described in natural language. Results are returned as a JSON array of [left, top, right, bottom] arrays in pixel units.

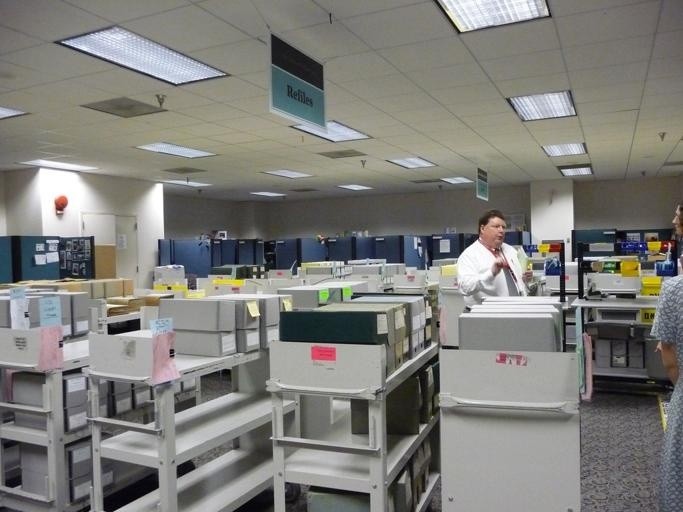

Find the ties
[[495, 250, 519, 296]]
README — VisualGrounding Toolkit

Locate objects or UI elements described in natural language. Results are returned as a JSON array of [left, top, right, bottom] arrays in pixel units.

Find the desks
[[571, 293, 683, 394]]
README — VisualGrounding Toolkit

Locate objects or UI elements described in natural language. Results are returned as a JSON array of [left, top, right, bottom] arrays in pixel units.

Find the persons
[[457, 211, 535, 317], [671, 203, 682, 238], [647, 275, 683, 511]]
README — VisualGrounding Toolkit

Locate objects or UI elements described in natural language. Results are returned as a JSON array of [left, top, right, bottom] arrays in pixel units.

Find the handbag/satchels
[[546, 256, 561, 275], [656, 260, 675, 276]]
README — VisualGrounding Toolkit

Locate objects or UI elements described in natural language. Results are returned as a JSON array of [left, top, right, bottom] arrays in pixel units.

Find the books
[[105, 295, 145, 313], [105, 303, 130, 317], [142, 294, 174, 306]]
